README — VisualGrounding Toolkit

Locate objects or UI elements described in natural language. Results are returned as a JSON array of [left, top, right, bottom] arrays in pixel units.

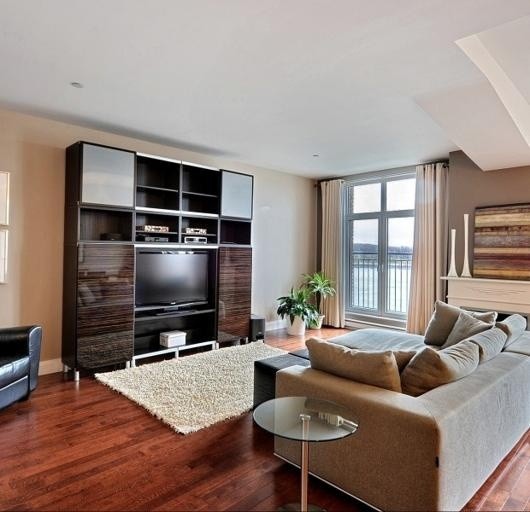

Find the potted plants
[[299, 271, 336, 329], [275, 286, 319, 336]]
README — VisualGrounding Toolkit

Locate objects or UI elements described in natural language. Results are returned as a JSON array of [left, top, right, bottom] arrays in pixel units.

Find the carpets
[[93, 339, 288, 436]]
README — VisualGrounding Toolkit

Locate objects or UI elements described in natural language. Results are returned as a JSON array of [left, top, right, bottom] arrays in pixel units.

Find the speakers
[[249, 315, 265, 341]]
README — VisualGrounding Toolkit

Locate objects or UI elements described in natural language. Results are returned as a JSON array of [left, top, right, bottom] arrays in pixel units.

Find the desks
[[440, 275, 530, 329]]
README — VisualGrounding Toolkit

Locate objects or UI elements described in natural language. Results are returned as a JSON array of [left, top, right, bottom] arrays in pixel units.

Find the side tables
[[252, 396, 360, 512]]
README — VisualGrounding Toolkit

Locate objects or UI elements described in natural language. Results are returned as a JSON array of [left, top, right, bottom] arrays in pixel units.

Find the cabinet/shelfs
[[61, 243, 136, 383], [62, 141, 254, 219], [76, 208, 253, 247], [216, 247, 252, 350]]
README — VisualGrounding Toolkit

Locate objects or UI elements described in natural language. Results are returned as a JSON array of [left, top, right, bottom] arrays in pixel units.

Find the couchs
[[274, 328, 530, 512]]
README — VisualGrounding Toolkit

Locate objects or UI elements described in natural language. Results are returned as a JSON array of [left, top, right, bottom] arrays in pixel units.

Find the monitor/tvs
[[134, 247, 213, 316]]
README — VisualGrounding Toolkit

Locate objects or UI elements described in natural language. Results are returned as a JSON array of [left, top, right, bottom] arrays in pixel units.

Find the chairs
[[0, 325, 42, 410]]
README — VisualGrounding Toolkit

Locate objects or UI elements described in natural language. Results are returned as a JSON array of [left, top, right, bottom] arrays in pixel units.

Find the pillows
[[306, 301, 527, 393]]
